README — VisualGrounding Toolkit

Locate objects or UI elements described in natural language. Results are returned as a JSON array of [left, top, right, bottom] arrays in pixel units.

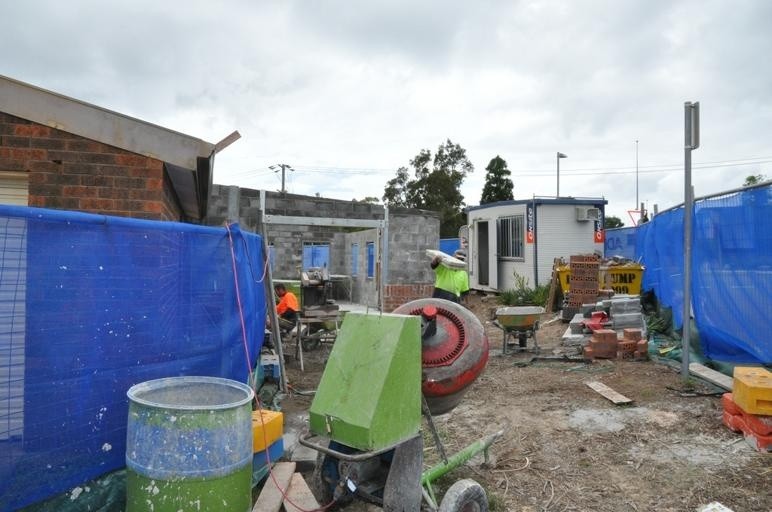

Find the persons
[[273, 282, 300, 324], [430, 248, 470, 304]]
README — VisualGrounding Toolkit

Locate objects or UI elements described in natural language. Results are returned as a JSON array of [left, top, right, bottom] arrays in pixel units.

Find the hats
[[455, 249, 466, 259]]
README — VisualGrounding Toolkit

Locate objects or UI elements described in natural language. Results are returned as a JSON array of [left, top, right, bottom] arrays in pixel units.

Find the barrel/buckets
[[123, 373, 257, 512], [273, 278, 302, 307]]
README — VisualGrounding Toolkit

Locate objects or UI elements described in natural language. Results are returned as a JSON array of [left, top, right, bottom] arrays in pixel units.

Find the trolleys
[[299, 306, 508, 512], [491, 306, 543, 353]]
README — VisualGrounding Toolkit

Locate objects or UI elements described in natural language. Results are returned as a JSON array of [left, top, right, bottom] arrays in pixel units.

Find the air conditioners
[[576, 207, 599, 221]]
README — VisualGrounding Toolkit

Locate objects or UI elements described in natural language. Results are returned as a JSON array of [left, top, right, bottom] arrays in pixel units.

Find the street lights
[[269, 162, 295, 191]]
